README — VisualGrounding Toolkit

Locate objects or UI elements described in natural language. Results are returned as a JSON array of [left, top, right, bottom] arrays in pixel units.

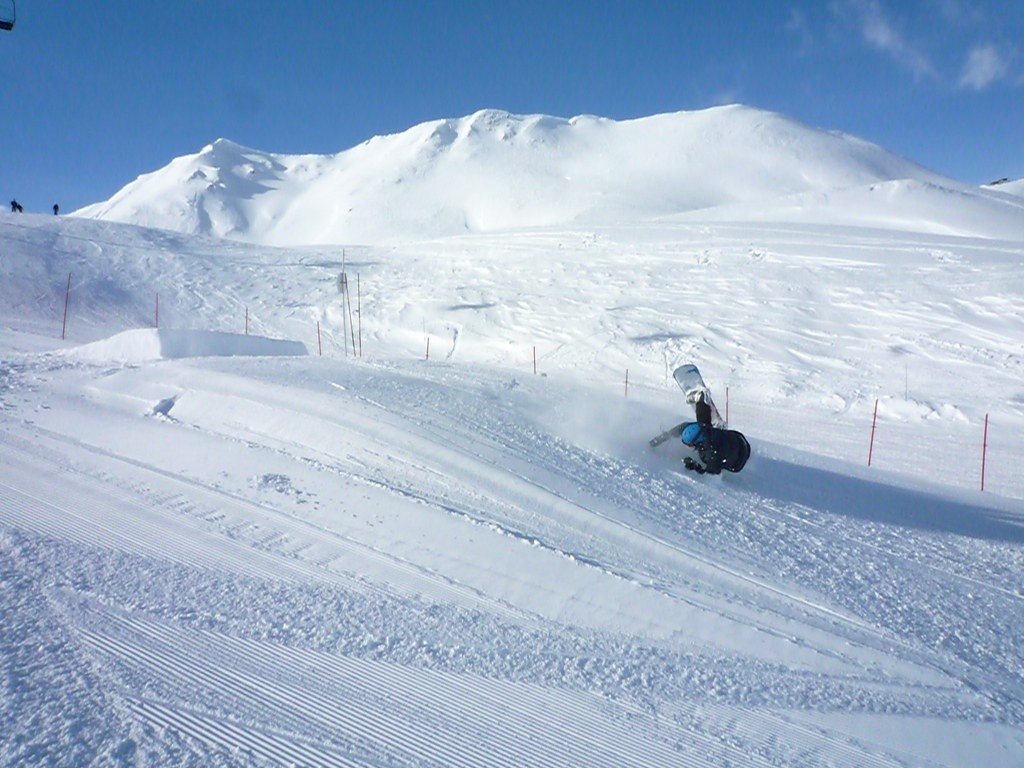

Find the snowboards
[[673, 364, 722, 428]]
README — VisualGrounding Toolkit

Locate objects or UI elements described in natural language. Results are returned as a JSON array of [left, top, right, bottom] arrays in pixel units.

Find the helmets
[[680, 424, 711, 447]]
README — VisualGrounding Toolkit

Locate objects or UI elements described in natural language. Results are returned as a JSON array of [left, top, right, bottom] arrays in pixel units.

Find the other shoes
[[685, 387, 708, 404]]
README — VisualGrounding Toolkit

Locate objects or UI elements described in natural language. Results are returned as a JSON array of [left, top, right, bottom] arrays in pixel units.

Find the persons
[[681, 388, 751, 476], [53, 203, 59, 215], [11, 199, 22, 213]]
